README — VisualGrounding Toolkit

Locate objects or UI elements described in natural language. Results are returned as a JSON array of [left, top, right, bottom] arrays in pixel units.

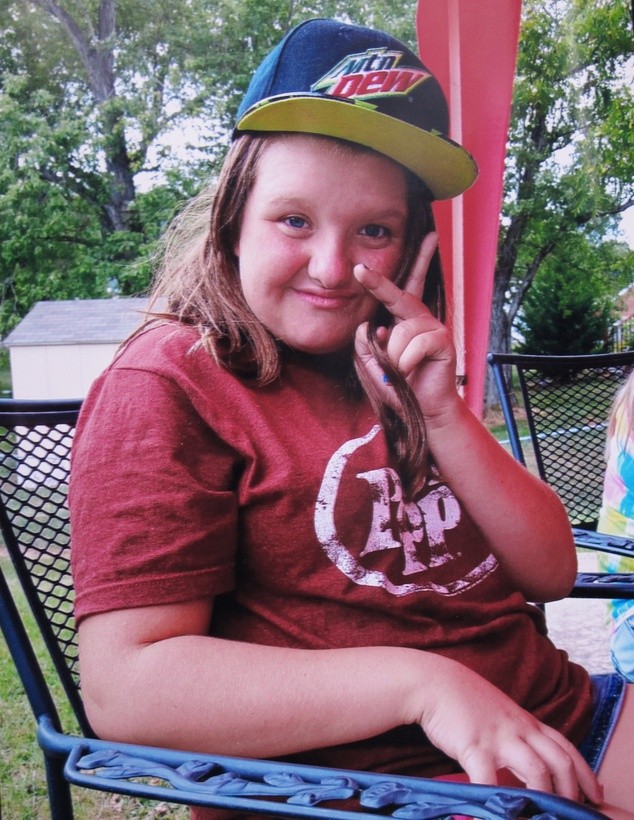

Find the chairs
[[0, 396, 633, 820], [486, 350, 634, 646]]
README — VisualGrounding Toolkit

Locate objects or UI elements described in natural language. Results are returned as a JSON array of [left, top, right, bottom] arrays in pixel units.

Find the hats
[[232, 18, 477, 204]]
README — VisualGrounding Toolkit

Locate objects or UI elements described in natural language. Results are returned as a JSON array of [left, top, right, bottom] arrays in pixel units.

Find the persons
[[66, 17, 634, 820], [594, 370, 634, 683]]
